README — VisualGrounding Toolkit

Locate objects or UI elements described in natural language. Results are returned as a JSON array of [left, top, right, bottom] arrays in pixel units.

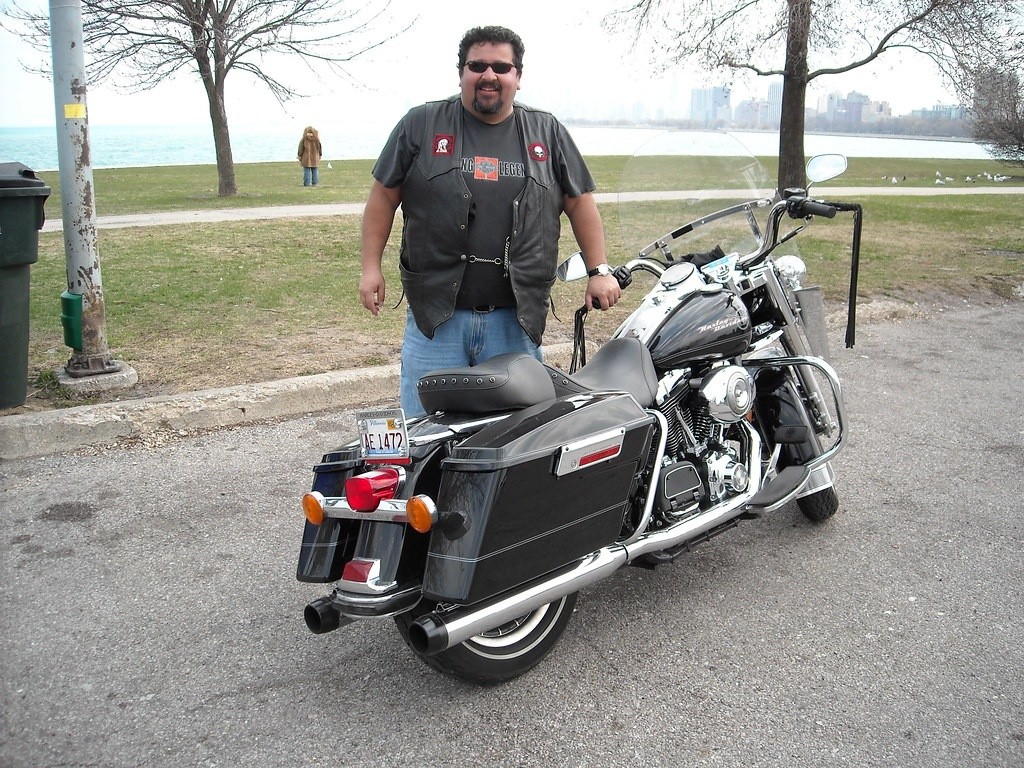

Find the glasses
[[462, 61, 517, 74]]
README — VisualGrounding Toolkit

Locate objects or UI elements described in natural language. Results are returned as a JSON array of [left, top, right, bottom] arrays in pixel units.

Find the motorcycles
[[295, 124, 864, 691]]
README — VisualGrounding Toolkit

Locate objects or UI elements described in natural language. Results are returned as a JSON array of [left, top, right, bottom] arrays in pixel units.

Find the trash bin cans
[[0, 161, 52, 411]]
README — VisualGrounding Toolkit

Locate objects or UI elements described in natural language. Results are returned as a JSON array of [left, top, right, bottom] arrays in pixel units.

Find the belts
[[470, 304, 506, 314]]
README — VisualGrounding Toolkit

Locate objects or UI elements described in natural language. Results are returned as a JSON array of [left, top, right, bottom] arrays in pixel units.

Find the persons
[[297, 126, 322, 188], [360, 26, 622, 422]]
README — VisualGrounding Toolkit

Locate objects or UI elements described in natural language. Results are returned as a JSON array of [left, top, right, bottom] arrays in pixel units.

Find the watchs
[[588, 263, 609, 278]]
